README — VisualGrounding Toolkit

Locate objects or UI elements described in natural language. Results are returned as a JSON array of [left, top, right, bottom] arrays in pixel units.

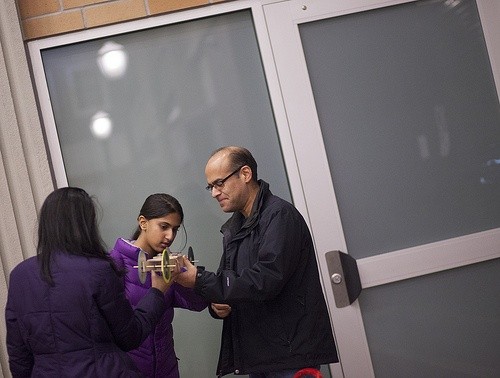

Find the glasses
[[205, 167, 244, 193]]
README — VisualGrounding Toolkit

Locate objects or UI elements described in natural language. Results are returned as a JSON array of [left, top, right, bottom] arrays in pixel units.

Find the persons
[[176, 147, 340, 378], [5, 186, 176, 378], [108, 193, 212, 378]]
